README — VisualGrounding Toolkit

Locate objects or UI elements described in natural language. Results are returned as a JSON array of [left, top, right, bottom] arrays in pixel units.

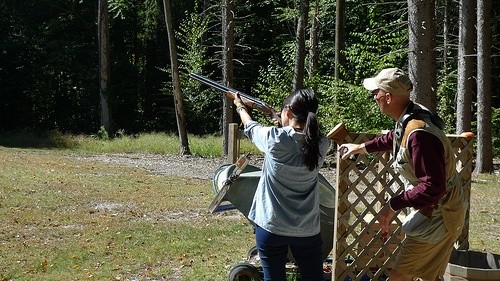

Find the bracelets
[[237, 106, 248, 113], [360, 143, 369, 156]]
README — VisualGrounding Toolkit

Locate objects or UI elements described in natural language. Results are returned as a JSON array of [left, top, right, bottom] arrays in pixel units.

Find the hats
[[364, 66, 413, 95]]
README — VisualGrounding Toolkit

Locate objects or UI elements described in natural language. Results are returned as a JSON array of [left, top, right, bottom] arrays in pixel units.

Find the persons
[[234, 88, 328, 281], [337, 68, 465, 281]]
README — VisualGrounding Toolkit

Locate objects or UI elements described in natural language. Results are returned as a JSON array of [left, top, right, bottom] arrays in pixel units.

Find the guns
[[189, 73, 282, 126]]
